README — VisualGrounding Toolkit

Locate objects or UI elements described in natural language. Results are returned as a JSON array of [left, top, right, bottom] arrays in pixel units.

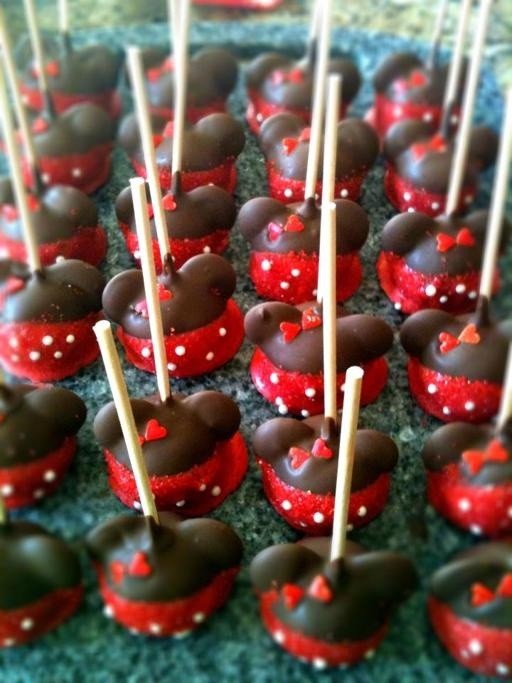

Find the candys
[[0, 1, 512, 678]]
